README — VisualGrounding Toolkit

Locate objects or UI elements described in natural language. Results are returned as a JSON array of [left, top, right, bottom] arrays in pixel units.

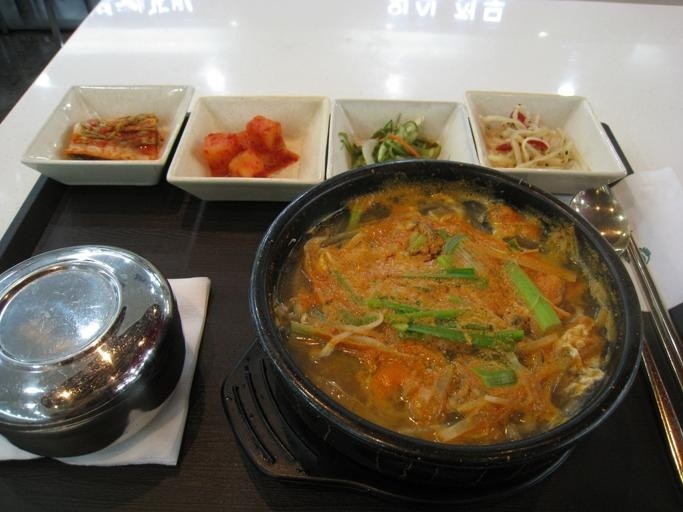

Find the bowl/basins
[[0, 244, 186, 459], [249, 160, 644, 476]]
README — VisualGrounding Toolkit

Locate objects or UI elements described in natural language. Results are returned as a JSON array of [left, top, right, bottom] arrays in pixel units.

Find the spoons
[[567, 186, 682, 482]]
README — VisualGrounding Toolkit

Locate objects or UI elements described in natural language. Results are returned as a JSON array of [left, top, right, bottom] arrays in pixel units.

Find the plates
[[166, 94, 331, 201], [462, 89, 627, 196], [325, 98, 482, 183], [18, 82, 193, 188]]
